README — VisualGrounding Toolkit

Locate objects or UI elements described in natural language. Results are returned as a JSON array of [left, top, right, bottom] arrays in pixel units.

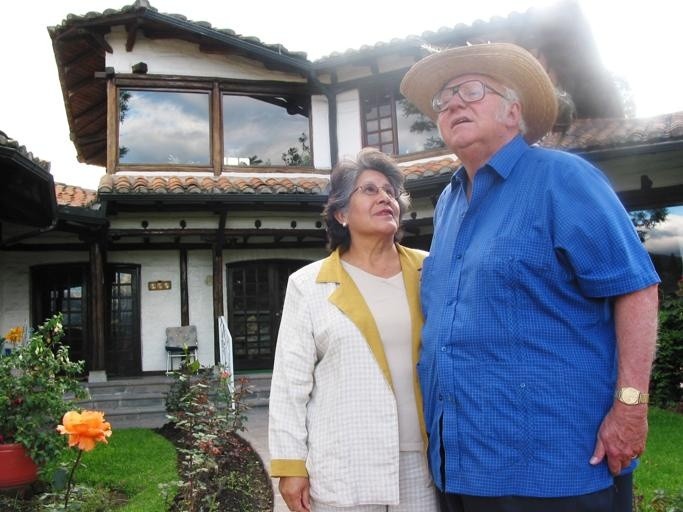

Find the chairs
[[166, 325, 200, 377]]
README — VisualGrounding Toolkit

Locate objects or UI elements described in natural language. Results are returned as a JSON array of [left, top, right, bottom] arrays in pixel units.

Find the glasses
[[431, 80, 509, 113], [348, 183, 395, 198]]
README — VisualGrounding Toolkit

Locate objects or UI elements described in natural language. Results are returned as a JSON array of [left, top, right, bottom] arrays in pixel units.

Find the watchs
[[611, 384, 651, 407]]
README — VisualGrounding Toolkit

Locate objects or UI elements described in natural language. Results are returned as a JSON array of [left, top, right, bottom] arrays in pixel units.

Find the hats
[[399, 42, 558, 146]]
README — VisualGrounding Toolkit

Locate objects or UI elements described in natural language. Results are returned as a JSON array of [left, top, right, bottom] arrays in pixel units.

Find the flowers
[[6, 326, 23, 344]]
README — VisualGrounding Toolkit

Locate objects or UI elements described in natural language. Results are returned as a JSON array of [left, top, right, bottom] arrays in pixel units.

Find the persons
[[400, 39, 663, 510], [266, 145, 439, 510]]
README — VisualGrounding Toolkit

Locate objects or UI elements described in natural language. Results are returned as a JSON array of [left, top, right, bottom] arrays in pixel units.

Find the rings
[[632, 454, 638, 460]]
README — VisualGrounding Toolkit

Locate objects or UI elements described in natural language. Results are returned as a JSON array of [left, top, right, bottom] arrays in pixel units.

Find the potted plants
[[0, 312, 92, 489]]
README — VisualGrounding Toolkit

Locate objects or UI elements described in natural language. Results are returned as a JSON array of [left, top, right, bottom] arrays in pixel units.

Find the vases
[[5, 348, 11, 356]]
